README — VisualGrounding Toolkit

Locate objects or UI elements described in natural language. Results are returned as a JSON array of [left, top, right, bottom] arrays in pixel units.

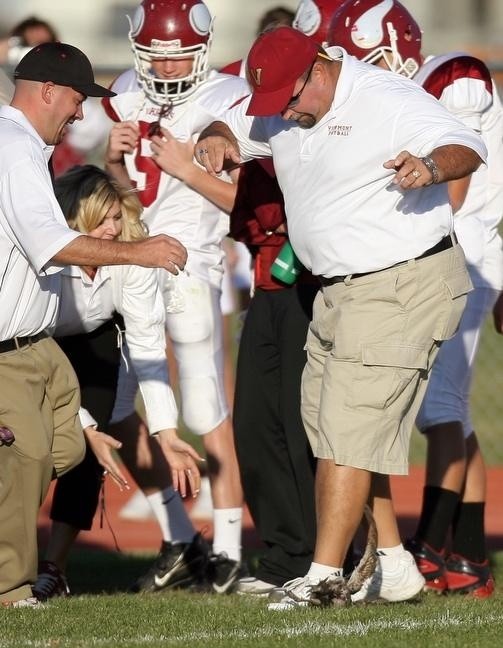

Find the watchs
[[418, 157, 438, 184]]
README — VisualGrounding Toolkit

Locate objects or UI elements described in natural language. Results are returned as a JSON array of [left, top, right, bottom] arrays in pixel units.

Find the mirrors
[[284, 503, 379, 609]]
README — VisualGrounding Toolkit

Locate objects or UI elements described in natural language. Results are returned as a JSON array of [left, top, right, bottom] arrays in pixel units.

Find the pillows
[[327, 0, 420, 78], [292, 0, 347, 48], [127, 0, 215, 106]]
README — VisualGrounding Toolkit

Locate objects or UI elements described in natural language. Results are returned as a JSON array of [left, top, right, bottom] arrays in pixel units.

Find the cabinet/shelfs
[[268, 237, 303, 286]]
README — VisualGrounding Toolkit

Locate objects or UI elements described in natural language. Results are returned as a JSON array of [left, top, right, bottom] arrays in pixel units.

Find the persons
[[328, 1, 500, 602], [101, 3, 252, 592], [229, 0, 352, 597], [0, 43, 187, 614], [221, 7, 294, 80], [2, 20, 106, 172], [194, 27, 487, 612], [119, 257, 233, 521], [31, 164, 206, 601]]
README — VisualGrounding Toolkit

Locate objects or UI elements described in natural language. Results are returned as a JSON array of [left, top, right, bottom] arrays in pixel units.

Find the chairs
[[30, 560, 71, 602]]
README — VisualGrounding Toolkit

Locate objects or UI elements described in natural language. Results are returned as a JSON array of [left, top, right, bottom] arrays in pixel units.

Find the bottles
[[14, 43, 117, 97], [244, 27, 317, 116]]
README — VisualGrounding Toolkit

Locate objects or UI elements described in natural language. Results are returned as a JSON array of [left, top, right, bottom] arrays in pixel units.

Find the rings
[[104, 470, 108, 475], [413, 170, 420, 177], [187, 469, 191, 474], [201, 150, 207, 153]]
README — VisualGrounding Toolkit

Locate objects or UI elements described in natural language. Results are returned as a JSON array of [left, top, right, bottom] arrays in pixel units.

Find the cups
[[287, 60, 315, 104]]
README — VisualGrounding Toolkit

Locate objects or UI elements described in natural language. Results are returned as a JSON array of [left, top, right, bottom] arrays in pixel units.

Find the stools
[[316, 231, 457, 283], [0, 328, 49, 352]]
